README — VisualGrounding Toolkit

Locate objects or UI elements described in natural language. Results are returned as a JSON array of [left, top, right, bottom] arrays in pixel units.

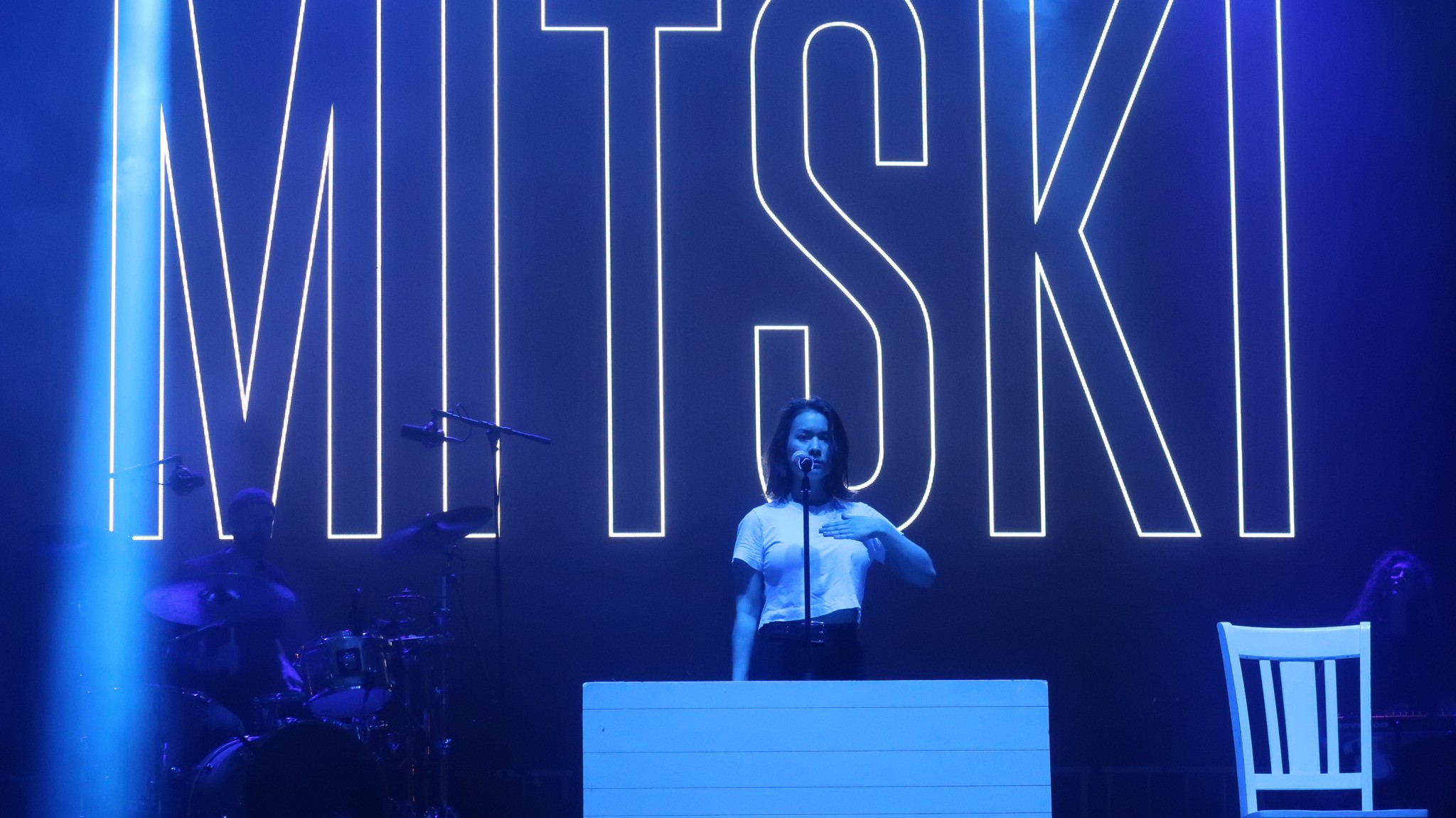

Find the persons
[[167, 489, 306, 738], [1326, 549, 1456, 818], [730, 400, 937, 681]]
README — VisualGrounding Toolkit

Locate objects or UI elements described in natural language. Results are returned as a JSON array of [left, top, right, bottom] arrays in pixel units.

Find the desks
[[580, 679, 1051, 818]]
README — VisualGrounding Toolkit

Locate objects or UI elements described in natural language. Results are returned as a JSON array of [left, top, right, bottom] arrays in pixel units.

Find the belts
[[759, 622, 858, 645]]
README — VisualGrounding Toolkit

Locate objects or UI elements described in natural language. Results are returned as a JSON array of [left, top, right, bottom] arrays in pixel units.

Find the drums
[[141, 678, 242, 797], [248, 688, 313, 731], [293, 626, 398, 723], [355, 711, 404, 755], [185, 717, 386, 818]]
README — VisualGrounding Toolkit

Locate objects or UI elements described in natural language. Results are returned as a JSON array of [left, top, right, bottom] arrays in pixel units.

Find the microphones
[[400, 425, 461, 446], [167, 473, 205, 493], [791, 450, 814, 472]]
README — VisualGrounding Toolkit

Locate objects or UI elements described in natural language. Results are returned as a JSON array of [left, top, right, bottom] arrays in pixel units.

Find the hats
[[227, 488, 273, 519]]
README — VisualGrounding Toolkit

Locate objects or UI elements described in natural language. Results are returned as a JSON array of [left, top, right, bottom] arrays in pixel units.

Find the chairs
[[1216, 621, 1429, 818]]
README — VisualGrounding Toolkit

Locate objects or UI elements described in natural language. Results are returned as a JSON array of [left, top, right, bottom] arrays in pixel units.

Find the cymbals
[[138, 577, 294, 627], [390, 506, 491, 550]]
